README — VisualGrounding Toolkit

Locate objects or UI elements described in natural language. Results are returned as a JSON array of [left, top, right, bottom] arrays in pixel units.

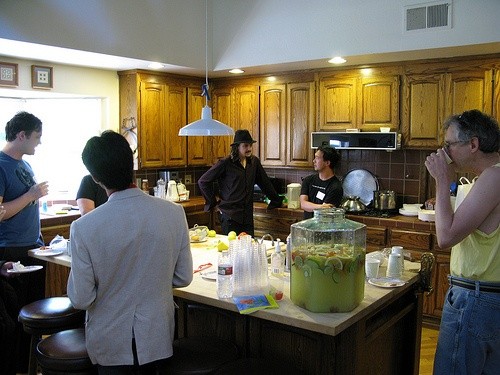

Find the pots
[[373, 190, 395, 210]]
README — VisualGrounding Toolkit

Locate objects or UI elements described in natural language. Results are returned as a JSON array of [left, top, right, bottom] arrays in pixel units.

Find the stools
[[18, 297, 96, 375], [158, 336, 307, 375]]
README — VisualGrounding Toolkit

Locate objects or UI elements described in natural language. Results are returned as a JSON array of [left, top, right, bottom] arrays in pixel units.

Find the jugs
[[287, 182, 302, 209], [166, 180, 179, 201]]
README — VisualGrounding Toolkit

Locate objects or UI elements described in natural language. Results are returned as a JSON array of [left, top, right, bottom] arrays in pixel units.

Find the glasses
[[444, 140, 463, 151]]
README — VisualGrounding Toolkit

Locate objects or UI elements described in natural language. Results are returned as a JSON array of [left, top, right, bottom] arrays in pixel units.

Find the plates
[[368, 278, 405, 288], [201, 268, 216, 280], [34, 249, 64, 256], [7, 266, 43, 273], [399, 204, 435, 223]]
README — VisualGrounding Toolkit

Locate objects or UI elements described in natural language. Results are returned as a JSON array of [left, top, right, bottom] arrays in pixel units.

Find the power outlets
[[185, 174, 191, 185]]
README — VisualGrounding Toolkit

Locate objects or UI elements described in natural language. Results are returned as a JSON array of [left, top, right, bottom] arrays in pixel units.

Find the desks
[[31, 230, 426, 375]]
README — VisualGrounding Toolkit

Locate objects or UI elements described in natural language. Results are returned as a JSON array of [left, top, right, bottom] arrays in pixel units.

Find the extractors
[[310, 129, 401, 153]]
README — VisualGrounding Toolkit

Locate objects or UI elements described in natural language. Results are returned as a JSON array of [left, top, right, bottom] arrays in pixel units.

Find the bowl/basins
[[253, 178, 291, 200]]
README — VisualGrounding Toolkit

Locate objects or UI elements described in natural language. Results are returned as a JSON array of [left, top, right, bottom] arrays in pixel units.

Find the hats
[[230, 129, 257, 146]]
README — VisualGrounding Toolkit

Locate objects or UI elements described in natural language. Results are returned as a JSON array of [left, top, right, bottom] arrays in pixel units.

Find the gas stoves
[[345, 205, 400, 220]]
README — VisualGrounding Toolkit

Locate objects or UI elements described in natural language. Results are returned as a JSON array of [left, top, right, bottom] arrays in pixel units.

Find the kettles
[[338, 195, 368, 214]]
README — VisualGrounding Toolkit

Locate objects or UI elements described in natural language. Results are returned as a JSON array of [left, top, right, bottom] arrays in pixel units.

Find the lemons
[[228, 231, 236, 241], [294, 256, 344, 274], [208, 230, 216, 236]]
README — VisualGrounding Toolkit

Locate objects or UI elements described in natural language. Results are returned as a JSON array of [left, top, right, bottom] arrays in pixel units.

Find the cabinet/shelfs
[[400, 58, 493, 148], [321, 67, 401, 131], [259, 74, 316, 168], [213, 76, 261, 166], [118, 70, 208, 170], [363, 225, 453, 318], [255, 212, 299, 244]]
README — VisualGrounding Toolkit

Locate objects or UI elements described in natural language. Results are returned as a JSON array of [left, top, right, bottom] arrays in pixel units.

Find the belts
[[448, 277, 500, 295]]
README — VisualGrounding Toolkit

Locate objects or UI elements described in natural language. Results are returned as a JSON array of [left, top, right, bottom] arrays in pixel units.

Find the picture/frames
[[0, 62, 18, 87], [32, 65, 53, 89]]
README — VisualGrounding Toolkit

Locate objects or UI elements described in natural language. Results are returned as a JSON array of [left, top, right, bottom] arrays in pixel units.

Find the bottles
[[287, 233, 305, 272], [157, 178, 165, 199], [290, 207, 367, 312], [218, 250, 233, 299], [142, 179, 149, 195]]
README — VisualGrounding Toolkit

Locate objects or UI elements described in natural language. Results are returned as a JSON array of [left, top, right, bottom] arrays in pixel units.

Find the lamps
[[178, 0, 235, 136]]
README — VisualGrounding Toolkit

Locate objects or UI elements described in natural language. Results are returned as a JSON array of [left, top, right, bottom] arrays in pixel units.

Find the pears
[[218, 240, 228, 251]]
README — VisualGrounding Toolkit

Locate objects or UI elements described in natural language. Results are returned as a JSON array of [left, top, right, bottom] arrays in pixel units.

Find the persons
[[299, 145, 343, 219], [199, 130, 287, 238], [67, 131, 193, 375], [0, 111, 48, 375], [425, 109, 500, 375], [76, 173, 110, 218]]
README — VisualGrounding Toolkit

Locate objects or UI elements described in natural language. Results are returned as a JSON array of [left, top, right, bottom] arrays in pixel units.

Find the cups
[[436, 148, 453, 164], [229, 234, 268, 293], [67, 239, 71, 257], [366, 259, 380, 279], [386, 245, 404, 279], [268, 276, 284, 301]]
